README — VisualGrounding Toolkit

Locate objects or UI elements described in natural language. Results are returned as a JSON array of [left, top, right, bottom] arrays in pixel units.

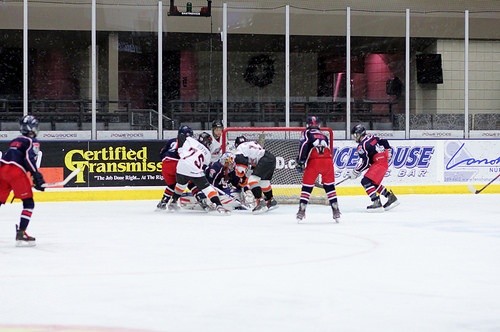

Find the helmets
[[235, 136, 246, 148], [212, 119, 223, 136], [178, 126, 193, 137], [20, 115, 39, 137], [306, 116, 321, 128], [351, 125, 366, 143], [199, 132, 212, 145]]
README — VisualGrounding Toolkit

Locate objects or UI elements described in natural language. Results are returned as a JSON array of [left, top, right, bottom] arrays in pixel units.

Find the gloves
[[296, 164, 305, 172], [349, 169, 360, 179], [33, 173, 45, 191]]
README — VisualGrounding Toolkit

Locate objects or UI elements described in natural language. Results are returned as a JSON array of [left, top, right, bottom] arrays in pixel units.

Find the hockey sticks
[[210, 184, 255, 210], [32, 168, 79, 187], [35, 151, 43, 168], [315, 165, 370, 189]]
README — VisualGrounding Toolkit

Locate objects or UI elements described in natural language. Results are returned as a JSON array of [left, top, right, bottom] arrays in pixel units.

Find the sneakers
[[297, 207, 305, 221], [252, 205, 263, 214], [168, 203, 180, 210], [217, 207, 230, 215], [333, 207, 342, 223], [15, 225, 36, 247], [383, 191, 400, 211], [266, 198, 279, 211], [157, 202, 166, 210], [203, 205, 210, 212], [367, 195, 383, 212]]
